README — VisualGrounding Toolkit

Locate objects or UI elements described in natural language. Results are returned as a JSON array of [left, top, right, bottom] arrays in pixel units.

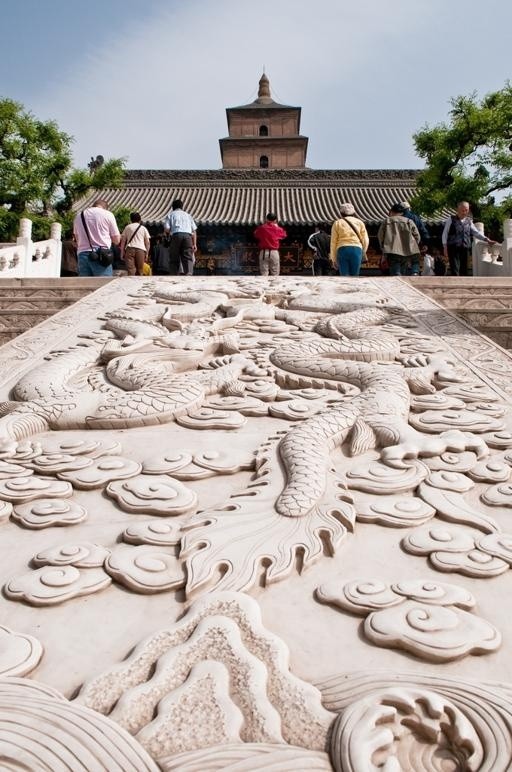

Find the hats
[[341, 204, 355, 215], [400, 201, 412, 210]]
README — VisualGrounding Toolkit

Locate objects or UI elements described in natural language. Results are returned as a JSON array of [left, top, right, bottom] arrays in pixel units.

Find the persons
[[440, 200, 498, 276], [110, 231, 170, 275], [120, 212, 151, 276], [419, 243, 435, 276], [162, 199, 198, 276], [396, 201, 430, 254], [376, 205, 421, 276], [306, 222, 331, 276], [251, 212, 287, 275], [329, 202, 370, 276], [72, 200, 122, 277]]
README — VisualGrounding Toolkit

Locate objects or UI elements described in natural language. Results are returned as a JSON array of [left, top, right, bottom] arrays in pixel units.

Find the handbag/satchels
[[97, 246, 113, 267], [162, 236, 171, 248]]
